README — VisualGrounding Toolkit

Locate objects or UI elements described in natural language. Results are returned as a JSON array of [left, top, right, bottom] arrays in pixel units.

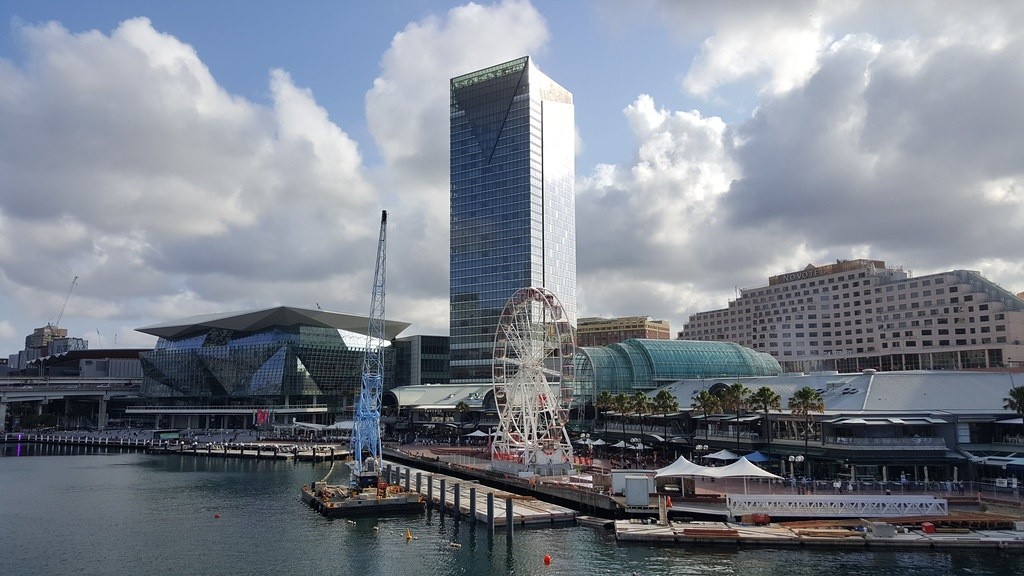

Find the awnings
[[653, 455, 786, 508]]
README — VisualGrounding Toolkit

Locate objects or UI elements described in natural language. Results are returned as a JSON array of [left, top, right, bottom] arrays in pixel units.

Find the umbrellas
[[461, 430, 503, 444], [571, 437, 653, 460], [701, 449, 772, 471]]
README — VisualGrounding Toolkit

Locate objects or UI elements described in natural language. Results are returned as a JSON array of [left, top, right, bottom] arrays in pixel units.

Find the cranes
[[46, 274, 80, 338]]
